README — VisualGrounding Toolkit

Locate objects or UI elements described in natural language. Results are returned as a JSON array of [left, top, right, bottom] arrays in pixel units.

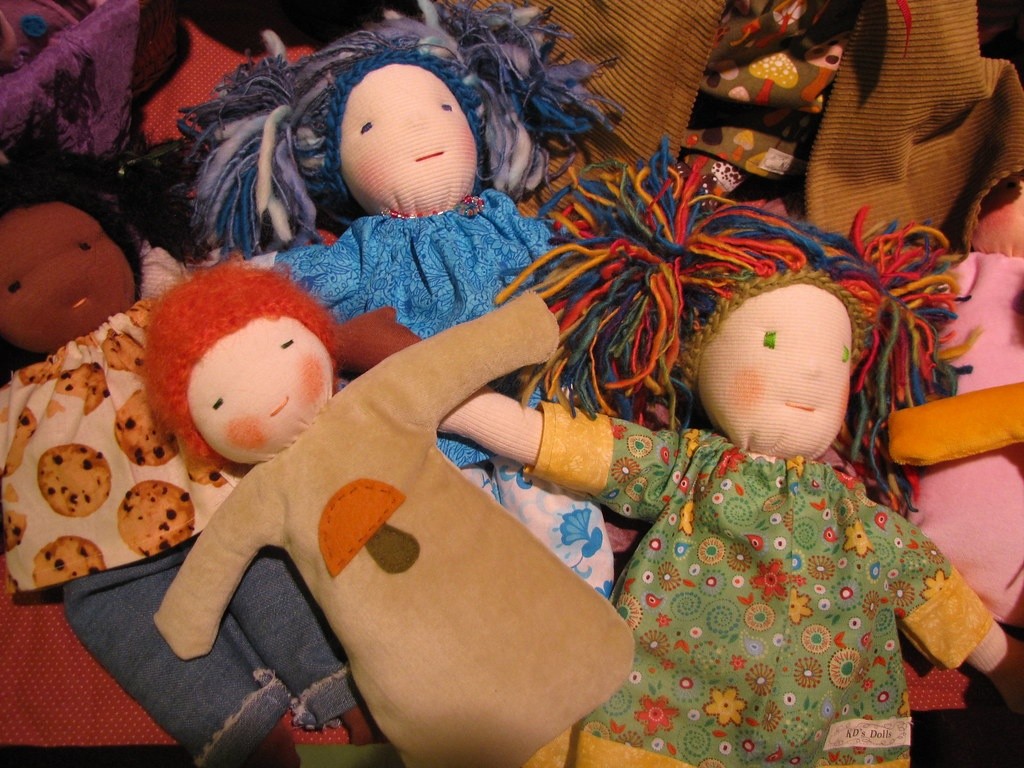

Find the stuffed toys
[[436, 139, 1024, 768], [145, 261, 641, 768], [0, 152, 390, 768], [0, 0, 1024, 599]]
